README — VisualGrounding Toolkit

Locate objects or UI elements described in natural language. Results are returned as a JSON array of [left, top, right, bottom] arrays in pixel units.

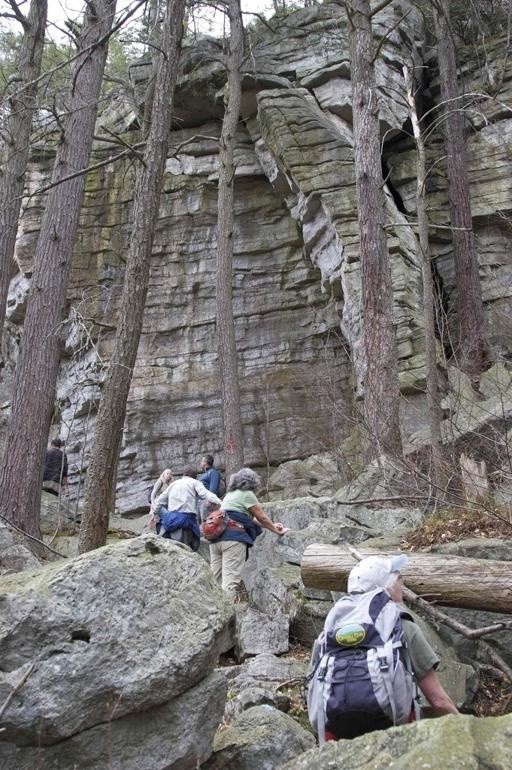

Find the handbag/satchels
[[148, 480, 163, 504], [207, 470, 226, 498], [204, 511, 230, 541]]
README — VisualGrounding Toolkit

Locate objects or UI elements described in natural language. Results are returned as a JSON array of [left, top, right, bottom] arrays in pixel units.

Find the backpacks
[[308, 589, 414, 739]]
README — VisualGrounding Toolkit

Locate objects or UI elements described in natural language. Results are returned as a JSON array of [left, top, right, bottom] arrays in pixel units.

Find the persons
[[306, 554, 460, 745], [208, 467, 292, 602], [43, 438, 68, 493], [198, 455, 220, 524], [153, 466, 222, 552], [149, 469, 173, 535]]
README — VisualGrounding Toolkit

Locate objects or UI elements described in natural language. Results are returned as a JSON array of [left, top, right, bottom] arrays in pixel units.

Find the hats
[[347, 553, 407, 596]]
